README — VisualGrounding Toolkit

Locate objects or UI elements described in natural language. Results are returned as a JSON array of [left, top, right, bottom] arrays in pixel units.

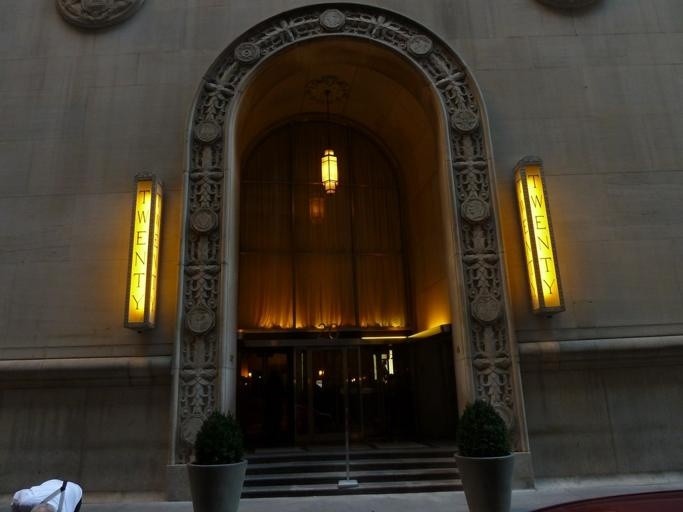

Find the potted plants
[[446, 395, 519, 510], [182, 407, 248, 512]]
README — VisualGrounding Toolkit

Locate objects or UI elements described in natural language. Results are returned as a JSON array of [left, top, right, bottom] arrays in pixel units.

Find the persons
[[10, 479, 84, 512]]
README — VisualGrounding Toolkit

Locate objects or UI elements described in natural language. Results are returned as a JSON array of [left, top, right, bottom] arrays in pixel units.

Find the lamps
[[304, 74, 355, 197], [121, 167, 165, 334], [510, 155, 564, 320]]
